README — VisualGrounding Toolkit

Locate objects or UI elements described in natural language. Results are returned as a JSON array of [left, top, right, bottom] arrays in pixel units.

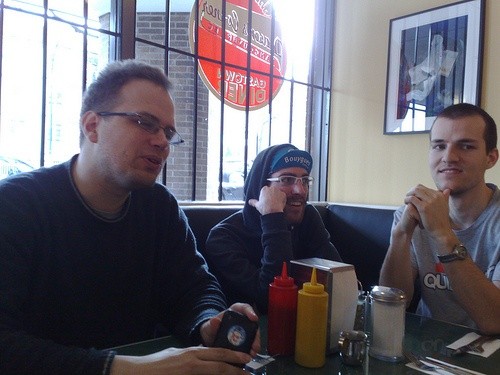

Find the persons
[[0, 60, 263, 374], [205, 143, 344, 332], [378, 103, 500, 336]]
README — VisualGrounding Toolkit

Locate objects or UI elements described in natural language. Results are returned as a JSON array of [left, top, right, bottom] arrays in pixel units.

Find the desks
[[100, 310, 500, 375]]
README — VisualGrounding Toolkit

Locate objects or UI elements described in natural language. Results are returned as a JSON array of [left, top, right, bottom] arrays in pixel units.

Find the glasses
[[265, 175, 315, 185], [96, 110, 185, 146]]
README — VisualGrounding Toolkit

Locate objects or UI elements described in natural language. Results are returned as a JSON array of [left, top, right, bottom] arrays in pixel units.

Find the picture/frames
[[384, 1, 486, 134]]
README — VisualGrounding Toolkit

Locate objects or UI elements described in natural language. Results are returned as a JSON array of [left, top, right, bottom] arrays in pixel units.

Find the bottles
[[266, 260, 298, 358], [294, 264, 329, 369]]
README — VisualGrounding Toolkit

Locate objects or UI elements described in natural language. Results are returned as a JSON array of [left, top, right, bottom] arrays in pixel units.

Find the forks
[[401, 351, 445, 375], [468, 336, 500, 353]]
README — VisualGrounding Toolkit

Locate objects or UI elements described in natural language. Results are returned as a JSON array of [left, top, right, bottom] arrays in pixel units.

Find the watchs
[[432, 241, 468, 264]]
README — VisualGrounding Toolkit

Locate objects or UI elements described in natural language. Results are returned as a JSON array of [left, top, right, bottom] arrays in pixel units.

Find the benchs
[[181, 203, 423, 314]]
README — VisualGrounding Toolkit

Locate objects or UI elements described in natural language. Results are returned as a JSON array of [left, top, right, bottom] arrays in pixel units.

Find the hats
[[265, 145, 313, 176]]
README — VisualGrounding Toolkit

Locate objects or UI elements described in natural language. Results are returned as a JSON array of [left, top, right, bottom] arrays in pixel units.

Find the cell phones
[[211, 310, 258, 369]]
